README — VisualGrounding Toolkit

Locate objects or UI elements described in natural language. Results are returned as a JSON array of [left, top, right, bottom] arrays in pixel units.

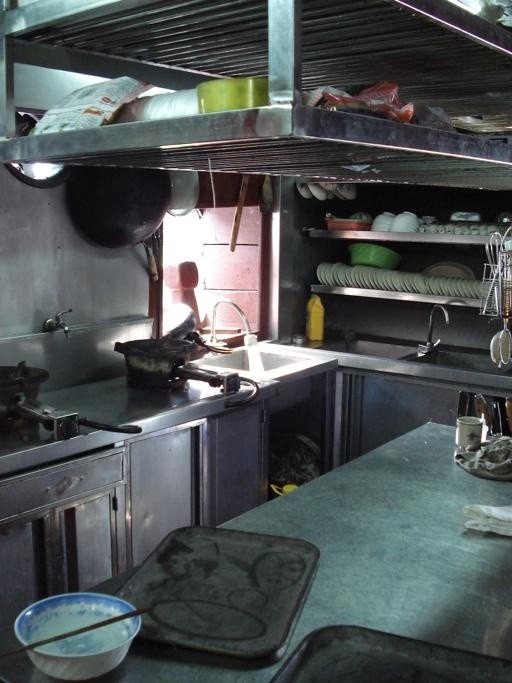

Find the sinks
[[400, 348, 512, 378], [189, 341, 339, 416]]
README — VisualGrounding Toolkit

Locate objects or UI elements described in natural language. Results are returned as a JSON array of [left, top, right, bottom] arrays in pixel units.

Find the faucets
[[42, 308, 74, 341], [425, 303, 450, 351], [210, 301, 259, 349]]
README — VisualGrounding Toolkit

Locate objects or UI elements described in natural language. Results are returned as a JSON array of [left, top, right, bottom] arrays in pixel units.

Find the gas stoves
[[114, 330, 242, 399], [0, 364, 80, 443]]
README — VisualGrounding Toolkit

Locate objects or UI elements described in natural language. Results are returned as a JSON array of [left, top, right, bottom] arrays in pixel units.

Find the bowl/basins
[[13, 590, 142, 683], [346, 243, 403, 269], [371, 209, 421, 234]]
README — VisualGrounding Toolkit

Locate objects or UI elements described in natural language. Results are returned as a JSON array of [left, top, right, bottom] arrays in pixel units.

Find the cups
[[425, 221, 504, 238], [456, 415, 482, 450]]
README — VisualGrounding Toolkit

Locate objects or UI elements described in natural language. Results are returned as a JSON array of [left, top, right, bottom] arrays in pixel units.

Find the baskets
[[324, 218, 369, 231]]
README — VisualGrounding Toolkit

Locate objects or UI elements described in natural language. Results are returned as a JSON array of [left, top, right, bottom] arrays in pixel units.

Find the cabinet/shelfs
[[1, 1, 512, 181], [0, 445, 127, 639], [309, 225, 512, 308], [130, 428, 193, 568]]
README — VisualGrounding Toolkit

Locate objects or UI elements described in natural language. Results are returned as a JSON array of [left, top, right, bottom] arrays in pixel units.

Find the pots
[[65, 141, 169, 282]]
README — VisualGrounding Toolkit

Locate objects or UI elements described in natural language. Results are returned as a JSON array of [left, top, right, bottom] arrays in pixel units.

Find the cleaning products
[[306, 294, 325, 341]]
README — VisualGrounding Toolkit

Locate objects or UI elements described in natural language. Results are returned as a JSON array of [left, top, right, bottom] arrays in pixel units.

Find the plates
[[314, 261, 490, 299]]
